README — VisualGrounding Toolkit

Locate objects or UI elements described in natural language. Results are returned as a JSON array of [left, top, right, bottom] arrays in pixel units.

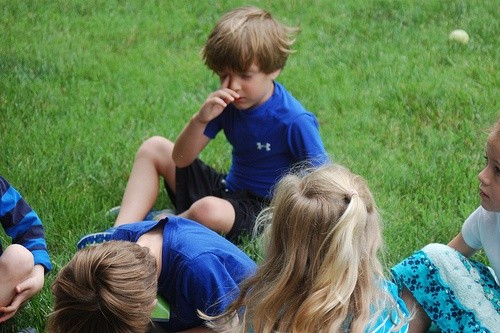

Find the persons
[[196, 162, 418, 332], [76, 5, 330, 251], [49, 215, 258, 332], [389, 121, 500, 333], [0, 175, 51, 323]]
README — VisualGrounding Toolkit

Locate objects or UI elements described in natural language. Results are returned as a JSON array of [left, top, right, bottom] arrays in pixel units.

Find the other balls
[[449, 29, 469, 44]]
